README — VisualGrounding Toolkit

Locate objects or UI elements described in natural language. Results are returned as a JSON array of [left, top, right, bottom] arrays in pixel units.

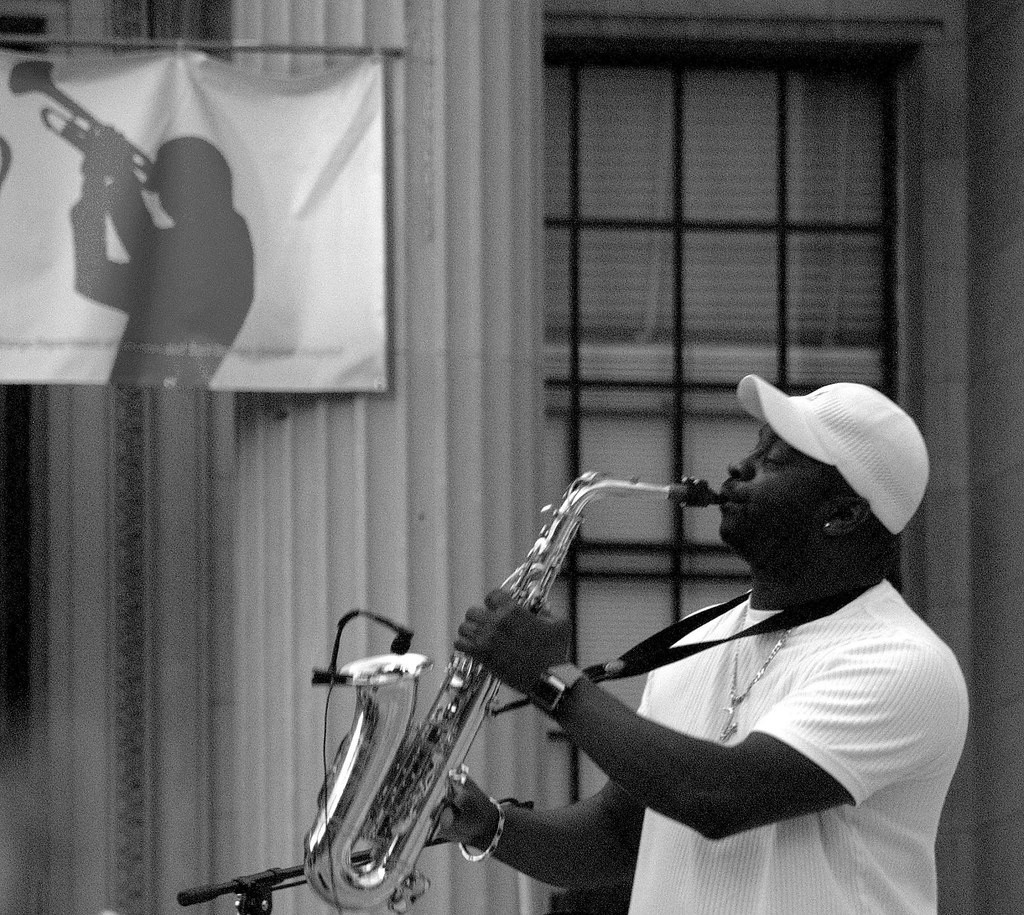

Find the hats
[[737, 374, 929, 535]]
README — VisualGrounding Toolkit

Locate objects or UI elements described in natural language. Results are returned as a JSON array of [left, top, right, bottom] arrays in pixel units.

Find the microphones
[[391, 630, 414, 655]]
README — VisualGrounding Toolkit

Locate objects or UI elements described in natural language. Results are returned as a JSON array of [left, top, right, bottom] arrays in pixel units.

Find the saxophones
[[302, 466, 732, 913]]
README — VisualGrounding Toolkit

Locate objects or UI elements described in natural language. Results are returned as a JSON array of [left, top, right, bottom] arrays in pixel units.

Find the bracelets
[[458, 797, 504, 864]]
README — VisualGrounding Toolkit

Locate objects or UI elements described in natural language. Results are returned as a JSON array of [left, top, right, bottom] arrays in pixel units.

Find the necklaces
[[715, 600, 798, 743]]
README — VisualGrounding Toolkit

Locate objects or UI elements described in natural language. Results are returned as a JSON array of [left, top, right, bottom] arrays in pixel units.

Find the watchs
[[528, 663, 588, 714]]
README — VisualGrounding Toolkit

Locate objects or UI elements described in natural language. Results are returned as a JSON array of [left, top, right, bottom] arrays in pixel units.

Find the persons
[[422, 375, 968, 915]]
